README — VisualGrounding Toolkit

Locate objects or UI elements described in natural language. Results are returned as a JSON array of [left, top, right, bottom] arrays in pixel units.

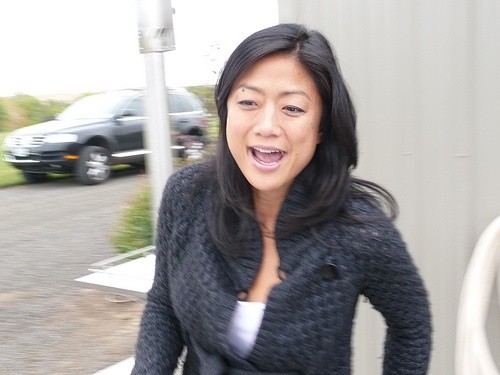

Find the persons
[[130, 22, 434, 375]]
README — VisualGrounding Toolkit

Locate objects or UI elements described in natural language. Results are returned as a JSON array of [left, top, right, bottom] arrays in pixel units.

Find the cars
[[0, 88, 213, 187]]
